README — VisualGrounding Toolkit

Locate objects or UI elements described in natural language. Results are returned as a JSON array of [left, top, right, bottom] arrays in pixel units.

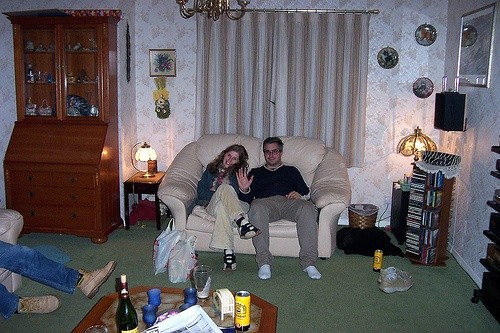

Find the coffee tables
[[69, 286, 279, 333]]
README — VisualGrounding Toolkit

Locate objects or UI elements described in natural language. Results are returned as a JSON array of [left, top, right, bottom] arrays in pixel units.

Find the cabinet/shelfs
[[404, 160, 461, 267], [470, 145, 500, 325], [2, 7, 124, 244]]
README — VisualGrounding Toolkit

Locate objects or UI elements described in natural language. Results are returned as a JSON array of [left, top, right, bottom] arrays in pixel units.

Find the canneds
[[235, 290, 251, 332], [373, 249, 383, 272]]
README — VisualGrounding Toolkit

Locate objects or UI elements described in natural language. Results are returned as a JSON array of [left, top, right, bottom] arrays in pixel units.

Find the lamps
[[130, 140, 157, 179], [396, 125, 438, 175], [175, 0, 251, 22]]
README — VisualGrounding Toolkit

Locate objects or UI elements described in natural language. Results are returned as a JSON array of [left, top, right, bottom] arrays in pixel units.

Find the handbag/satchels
[[167, 236, 199, 283], [153, 218, 184, 275]]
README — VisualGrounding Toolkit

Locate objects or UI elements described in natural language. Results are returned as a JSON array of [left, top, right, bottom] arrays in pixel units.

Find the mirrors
[[456, 1, 499, 89]]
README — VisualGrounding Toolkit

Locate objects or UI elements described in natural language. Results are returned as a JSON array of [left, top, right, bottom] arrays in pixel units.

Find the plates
[[461, 25, 478, 47], [414, 23, 438, 46], [412, 77, 435, 99], [377, 47, 400, 69]]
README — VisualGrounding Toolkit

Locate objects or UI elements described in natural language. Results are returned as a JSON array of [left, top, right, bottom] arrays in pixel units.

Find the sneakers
[[18, 294, 60, 314], [380, 272, 414, 293], [76, 260, 117, 299], [378, 267, 412, 282], [224, 249, 236, 270], [237, 218, 263, 238]]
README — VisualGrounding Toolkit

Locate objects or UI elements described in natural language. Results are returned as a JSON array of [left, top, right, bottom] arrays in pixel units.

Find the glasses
[[264, 149, 283, 155]]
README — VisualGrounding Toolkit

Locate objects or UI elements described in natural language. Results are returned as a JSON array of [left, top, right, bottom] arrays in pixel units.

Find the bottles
[[114, 274, 139, 333]]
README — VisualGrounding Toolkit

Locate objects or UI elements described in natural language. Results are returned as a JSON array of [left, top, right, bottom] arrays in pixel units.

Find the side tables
[[389, 182, 410, 245], [124, 170, 171, 232]]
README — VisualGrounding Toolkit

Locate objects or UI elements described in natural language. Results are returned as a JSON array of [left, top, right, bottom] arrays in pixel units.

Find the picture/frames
[[149, 48, 176, 77]]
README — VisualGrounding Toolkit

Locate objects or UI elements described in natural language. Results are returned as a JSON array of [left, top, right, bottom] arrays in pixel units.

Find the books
[[405, 169, 427, 255], [422, 247, 435, 264], [422, 209, 440, 228], [423, 229, 438, 246], [428, 172, 444, 188], [427, 189, 442, 207]]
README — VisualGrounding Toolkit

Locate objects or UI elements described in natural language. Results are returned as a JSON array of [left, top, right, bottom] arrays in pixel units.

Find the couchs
[[0, 207, 24, 293], [156, 133, 352, 261]]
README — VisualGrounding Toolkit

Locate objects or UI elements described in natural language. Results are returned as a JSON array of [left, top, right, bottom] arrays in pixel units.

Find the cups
[[183, 287, 197, 305], [25, 41, 99, 117], [193, 265, 212, 302], [141, 304, 157, 328], [147, 288, 161, 307]]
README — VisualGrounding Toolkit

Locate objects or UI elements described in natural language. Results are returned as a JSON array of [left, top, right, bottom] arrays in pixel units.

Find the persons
[[198, 145, 262, 271], [0, 240, 117, 320], [236, 137, 321, 279]]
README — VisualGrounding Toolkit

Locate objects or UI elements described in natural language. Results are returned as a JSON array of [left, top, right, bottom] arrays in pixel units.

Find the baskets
[[348, 204, 378, 228]]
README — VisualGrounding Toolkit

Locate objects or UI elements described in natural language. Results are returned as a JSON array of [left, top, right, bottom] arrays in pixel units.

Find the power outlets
[[384, 197, 391, 205]]
